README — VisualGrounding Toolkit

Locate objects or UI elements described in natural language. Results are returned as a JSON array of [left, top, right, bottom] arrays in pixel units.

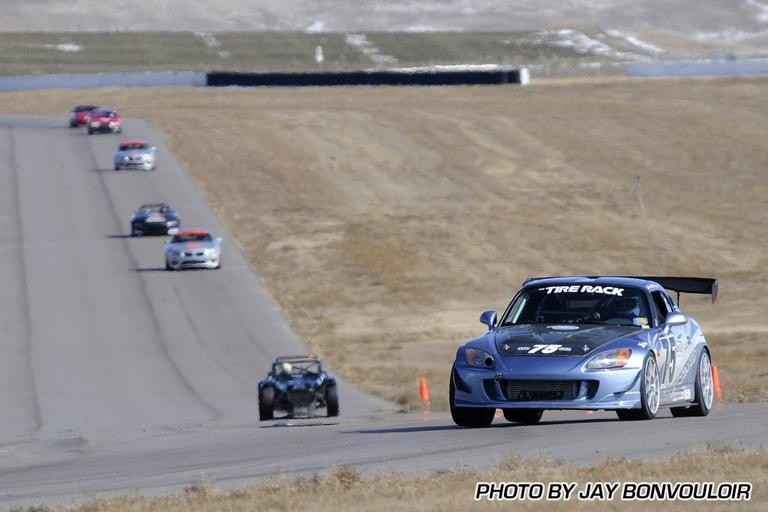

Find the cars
[[450, 274, 719, 427], [87, 108, 122, 134], [164, 229, 224, 271], [113, 142, 157, 171], [130, 202, 181, 236], [70, 105, 98, 127]]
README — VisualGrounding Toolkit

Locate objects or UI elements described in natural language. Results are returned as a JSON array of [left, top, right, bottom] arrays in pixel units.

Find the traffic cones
[[418, 376, 429, 400], [712, 365, 720, 398]]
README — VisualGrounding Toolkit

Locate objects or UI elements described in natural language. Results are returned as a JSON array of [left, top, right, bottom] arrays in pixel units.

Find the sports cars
[[258, 354, 340, 422]]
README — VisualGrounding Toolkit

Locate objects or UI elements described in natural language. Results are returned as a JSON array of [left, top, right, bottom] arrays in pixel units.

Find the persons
[[279, 361, 294, 377]]
